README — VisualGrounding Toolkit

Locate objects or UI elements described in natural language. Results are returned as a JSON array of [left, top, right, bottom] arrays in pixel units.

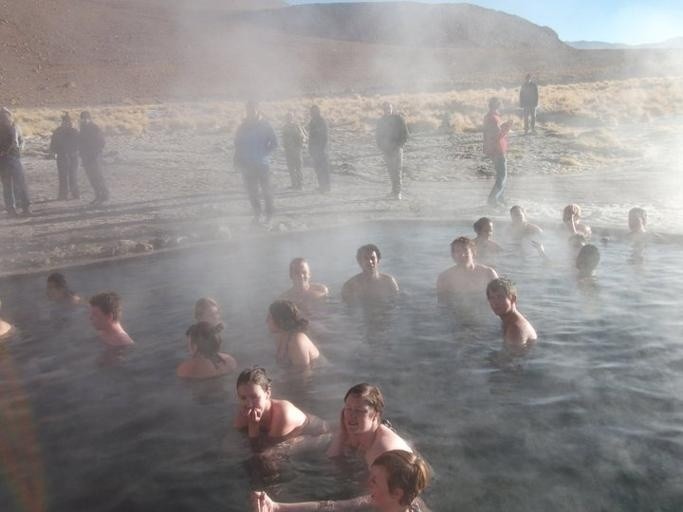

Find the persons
[[0, 113, 32, 218], [326, 383, 413, 470], [503, 205, 542, 239], [470, 216, 503, 254], [482, 98, 514, 206], [281, 112, 305, 191], [0, 319, 11, 336], [436, 236, 499, 293], [78, 111, 108, 206], [264, 300, 319, 368], [527, 240, 600, 287], [0, 107, 24, 215], [233, 366, 332, 486], [176, 321, 236, 379], [278, 257, 327, 299], [559, 204, 591, 239], [375, 101, 409, 201], [566, 233, 588, 255], [87, 290, 133, 346], [46, 272, 79, 305], [193, 297, 219, 324], [519, 74, 538, 132], [50, 115, 78, 201], [304, 105, 330, 194], [233, 103, 279, 229], [341, 244, 399, 299], [486, 278, 538, 346], [248, 449, 433, 511], [627, 207, 649, 240]]
